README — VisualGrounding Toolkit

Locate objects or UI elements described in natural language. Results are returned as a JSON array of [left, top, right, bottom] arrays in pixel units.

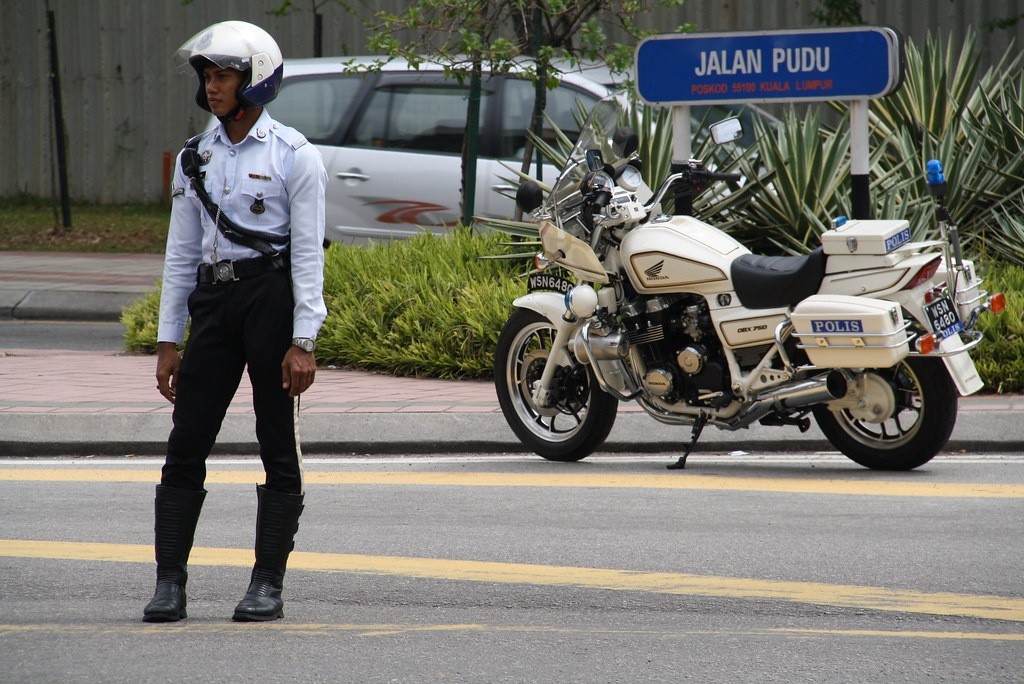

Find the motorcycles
[[495, 90, 1006, 471]]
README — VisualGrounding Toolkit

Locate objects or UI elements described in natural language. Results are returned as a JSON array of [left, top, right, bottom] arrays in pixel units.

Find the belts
[[197, 249, 290, 284]]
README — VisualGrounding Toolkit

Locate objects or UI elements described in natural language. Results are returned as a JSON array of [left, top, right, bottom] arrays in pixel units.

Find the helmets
[[188, 21, 284, 113]]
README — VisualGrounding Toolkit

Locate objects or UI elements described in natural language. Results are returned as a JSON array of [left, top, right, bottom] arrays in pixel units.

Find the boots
[[233, 485, 306, 622], [142, 484, 208, 621]]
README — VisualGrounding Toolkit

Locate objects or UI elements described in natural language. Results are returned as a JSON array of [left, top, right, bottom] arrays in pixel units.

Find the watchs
[[293, 338, 316, 351]]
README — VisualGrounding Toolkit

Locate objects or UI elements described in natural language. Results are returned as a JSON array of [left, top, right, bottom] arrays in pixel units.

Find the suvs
[[205, 57, 783, 250]]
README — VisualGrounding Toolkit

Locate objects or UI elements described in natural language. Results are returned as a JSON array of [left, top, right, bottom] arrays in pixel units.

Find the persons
[[143, 20, 329, 619]]
